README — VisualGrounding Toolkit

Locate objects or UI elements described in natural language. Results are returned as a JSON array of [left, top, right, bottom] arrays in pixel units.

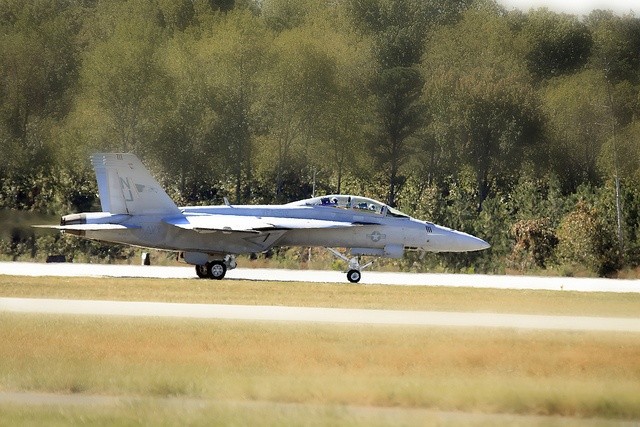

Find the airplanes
[[30, 152, 491, 282]]
